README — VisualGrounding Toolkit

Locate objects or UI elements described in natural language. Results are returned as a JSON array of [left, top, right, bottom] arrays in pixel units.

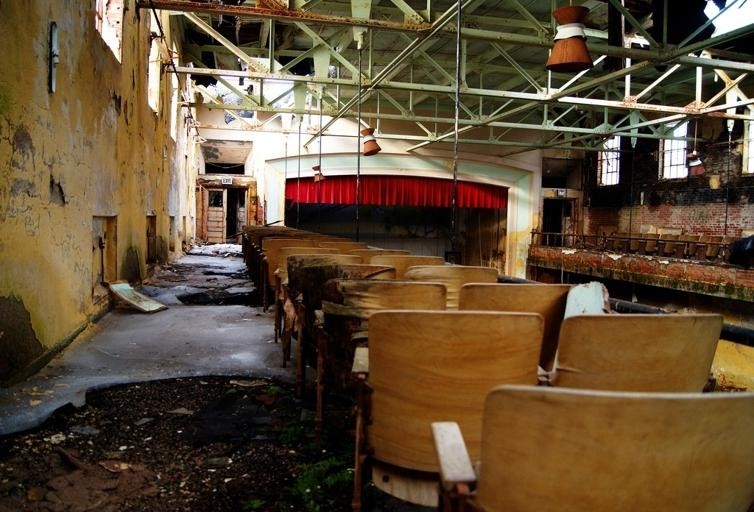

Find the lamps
[[541, 0, 593, 73], [311, 139, 325, 183], [359, 87, 382, 157]]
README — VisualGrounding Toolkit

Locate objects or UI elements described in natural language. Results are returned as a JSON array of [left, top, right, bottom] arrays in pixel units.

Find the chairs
[[240, 221, 754, 512]]
